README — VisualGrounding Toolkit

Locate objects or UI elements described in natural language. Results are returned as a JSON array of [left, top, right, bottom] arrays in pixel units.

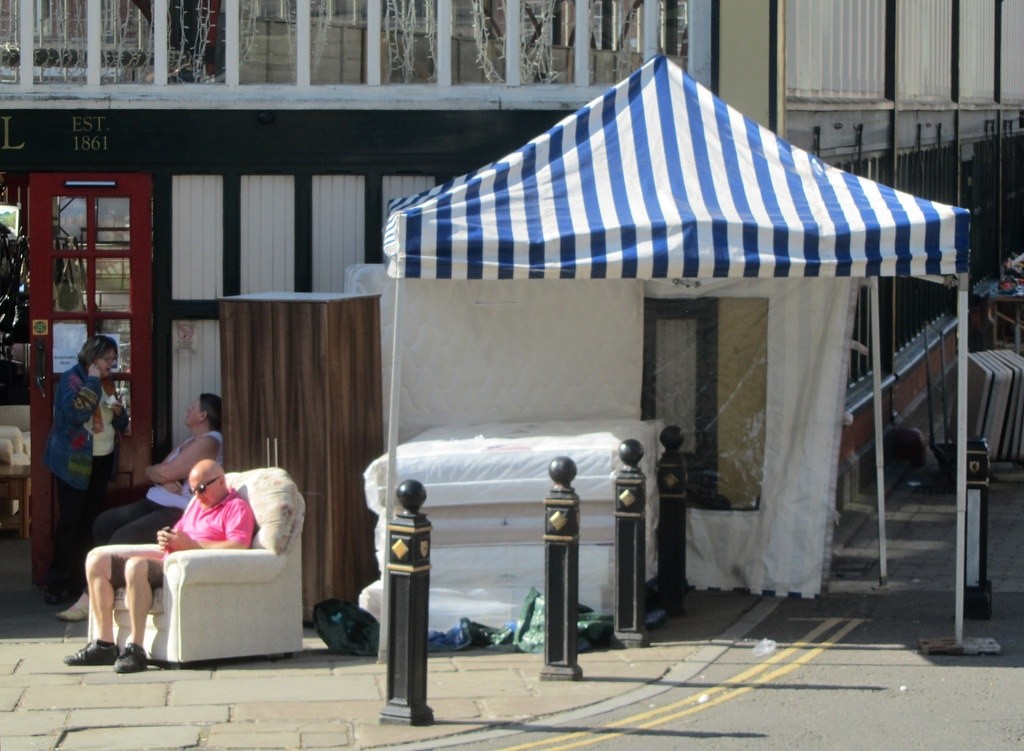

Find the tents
[[380, 51, 978, 667]]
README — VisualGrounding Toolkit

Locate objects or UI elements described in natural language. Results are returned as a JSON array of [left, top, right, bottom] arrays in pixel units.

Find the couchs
[[85, 464, 306, 670]]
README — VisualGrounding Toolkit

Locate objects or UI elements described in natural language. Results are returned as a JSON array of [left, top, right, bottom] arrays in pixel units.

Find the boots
[[56, 593, 89, 621]]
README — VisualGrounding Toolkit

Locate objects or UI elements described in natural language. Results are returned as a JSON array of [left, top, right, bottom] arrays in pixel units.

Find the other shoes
[[45, 586, 60, 605]]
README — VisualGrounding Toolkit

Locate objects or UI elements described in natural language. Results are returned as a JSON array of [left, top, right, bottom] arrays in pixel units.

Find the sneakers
[[114, 644, 148, 673], [63, 640, 117, 665]]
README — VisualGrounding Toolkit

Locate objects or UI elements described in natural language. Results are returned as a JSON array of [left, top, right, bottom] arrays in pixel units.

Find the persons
[[65, 458, 257, 673], [131, 0, 219, 84], [57, 392, 225, 624], [46, 334, 127, 608]]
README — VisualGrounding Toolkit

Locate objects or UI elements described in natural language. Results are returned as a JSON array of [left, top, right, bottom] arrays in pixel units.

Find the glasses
[[191, 476, 220, 494]]
